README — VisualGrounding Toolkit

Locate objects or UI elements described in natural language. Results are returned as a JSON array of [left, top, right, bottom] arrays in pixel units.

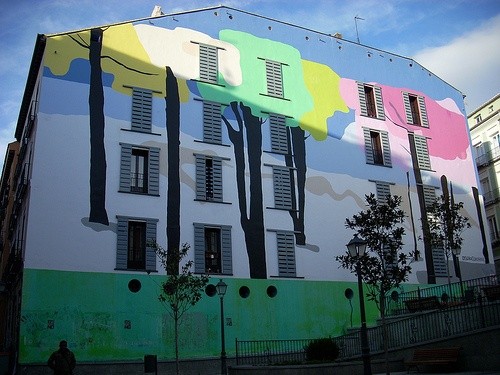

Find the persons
[[441, 291, 449, 304], [47, 340, 77, 375]]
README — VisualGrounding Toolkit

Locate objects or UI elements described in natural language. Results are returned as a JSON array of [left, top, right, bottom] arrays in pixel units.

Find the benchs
[[480, 285, 500, 300], [404, 347, 458, 373], [403, 299, 427, 313]]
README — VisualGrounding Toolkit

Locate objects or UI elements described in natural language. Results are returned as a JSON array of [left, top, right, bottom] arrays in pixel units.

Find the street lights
[[346, 234, 373, 375], [215, 279, 229, 375]]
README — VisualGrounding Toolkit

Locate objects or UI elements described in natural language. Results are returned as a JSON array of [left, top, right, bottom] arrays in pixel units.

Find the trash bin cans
[[144, 354, 157, 375]]
